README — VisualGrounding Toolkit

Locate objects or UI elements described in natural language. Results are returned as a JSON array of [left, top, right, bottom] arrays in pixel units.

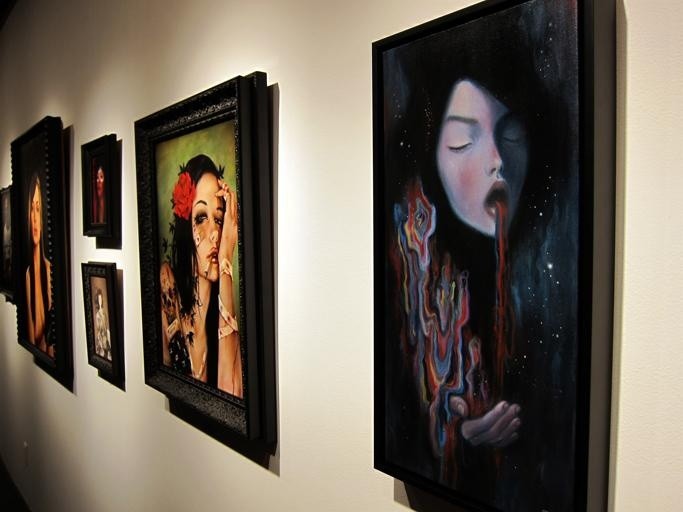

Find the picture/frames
[[82, 263, 121, 378], [9, 115, 74, 370], [81, 133, 116, 237], [134, 71, 277, 442], [0, 184, 15, 303]]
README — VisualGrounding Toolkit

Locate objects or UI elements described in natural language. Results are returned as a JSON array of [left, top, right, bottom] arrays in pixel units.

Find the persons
[[386, 53, 562, 492], [158, 150, 245, 399], [20, 171, 58, 361], [90, 285, 109, 361], [87, 161, 105, 226]]
